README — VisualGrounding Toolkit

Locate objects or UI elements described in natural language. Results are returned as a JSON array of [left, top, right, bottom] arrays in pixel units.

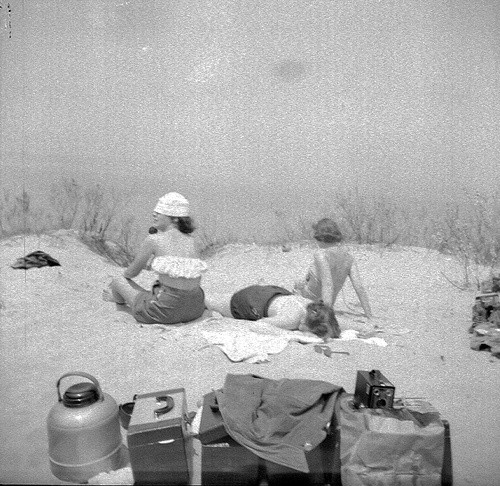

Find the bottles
[[48, 372, 123, 484]]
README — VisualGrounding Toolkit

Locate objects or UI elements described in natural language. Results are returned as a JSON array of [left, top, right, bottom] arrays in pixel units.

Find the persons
[[204, 285, 341, 340], [102, 192, 207, 324], [292, 218, 386, 328]]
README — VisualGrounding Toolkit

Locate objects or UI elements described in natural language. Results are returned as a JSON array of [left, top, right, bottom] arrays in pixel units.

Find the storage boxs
[[126, 388, 456, 486]]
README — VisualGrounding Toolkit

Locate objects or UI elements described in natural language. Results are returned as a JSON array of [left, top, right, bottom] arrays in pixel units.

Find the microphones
[[148, 226, 157, 234]]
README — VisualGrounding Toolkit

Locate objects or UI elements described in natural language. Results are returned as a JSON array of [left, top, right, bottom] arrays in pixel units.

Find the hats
[[154, 191, 190, 217]]
